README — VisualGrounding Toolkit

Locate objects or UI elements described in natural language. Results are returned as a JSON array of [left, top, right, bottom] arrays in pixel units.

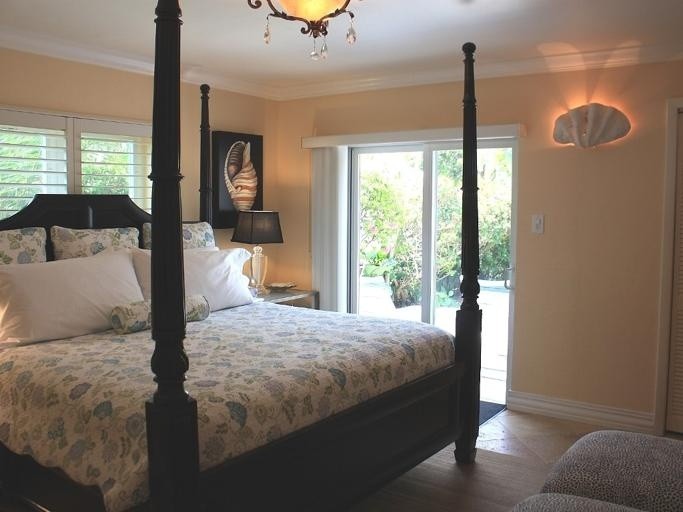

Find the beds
[[1, 193, 483, 510]]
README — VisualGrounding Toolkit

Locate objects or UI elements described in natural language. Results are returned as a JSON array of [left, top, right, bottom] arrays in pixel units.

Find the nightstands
[[255, 287, 320, 311]]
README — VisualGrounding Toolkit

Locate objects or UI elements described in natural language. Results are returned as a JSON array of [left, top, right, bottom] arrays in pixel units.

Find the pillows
[[1, 220, 256, 348]]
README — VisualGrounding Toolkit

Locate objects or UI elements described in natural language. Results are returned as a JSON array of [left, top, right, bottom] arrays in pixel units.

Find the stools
[[506, 428, 682, 512]]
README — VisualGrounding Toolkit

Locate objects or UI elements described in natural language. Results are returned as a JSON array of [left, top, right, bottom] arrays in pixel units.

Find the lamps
[[247, 0, 359, 62], [551, 101, 631, 151], [229, 208, 284, 297]]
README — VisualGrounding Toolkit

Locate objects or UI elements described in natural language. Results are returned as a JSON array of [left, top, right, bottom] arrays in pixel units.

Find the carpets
[[330, 400, 507, 510]]
